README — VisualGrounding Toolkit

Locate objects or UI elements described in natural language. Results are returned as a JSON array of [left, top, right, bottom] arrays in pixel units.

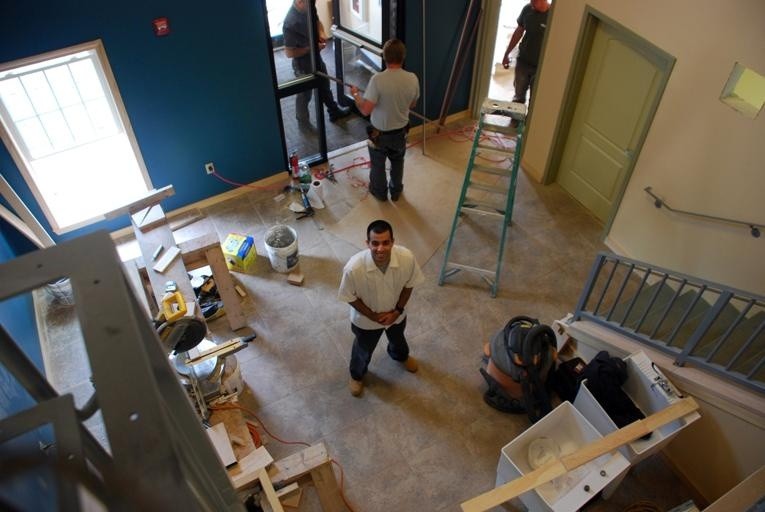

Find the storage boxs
[[220, 233, 257, 273]]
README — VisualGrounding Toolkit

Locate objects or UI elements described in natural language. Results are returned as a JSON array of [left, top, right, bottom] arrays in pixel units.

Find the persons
[[350, 38, 421, 201], [502, 0, 551, 128], [337, 220, 425, 397], [283, 0, 351, 135]]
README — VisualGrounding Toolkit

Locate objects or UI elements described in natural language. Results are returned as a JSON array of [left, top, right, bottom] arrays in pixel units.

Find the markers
[[151, 244, 164, 262]]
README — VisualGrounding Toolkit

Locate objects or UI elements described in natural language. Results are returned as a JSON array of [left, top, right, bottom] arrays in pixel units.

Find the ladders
[[436, 97, 530, 298]]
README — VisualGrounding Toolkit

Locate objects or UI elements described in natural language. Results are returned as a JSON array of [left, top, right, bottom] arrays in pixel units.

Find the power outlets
[[205, 163, 214, 175]]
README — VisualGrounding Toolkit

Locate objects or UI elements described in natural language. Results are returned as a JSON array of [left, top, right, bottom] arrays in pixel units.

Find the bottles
[[299, 161, 312, 192], [290, 152, 299, 179]]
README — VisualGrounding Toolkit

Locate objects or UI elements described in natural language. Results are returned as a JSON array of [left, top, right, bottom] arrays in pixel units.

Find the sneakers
[[391, 184, 403, 201], [369, 182, 387, 202], [329, 107, 350, 123], [351, 378, 362, 396], [299, 121, 315, 135], [406, 358, 417, 374]]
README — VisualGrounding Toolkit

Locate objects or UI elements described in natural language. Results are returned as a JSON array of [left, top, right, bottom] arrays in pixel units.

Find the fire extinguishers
[[289, 149, 299, 180]]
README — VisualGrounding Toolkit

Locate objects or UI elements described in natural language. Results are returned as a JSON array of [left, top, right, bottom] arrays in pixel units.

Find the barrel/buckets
[[46, 278, 75, 306], [222, 353, 245, 397], [264, 225, 300, 273]]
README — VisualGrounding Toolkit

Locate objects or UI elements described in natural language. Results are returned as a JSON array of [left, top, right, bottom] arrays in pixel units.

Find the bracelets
[[352, 92, 358, 96], [396, 304, 405, 315]]
